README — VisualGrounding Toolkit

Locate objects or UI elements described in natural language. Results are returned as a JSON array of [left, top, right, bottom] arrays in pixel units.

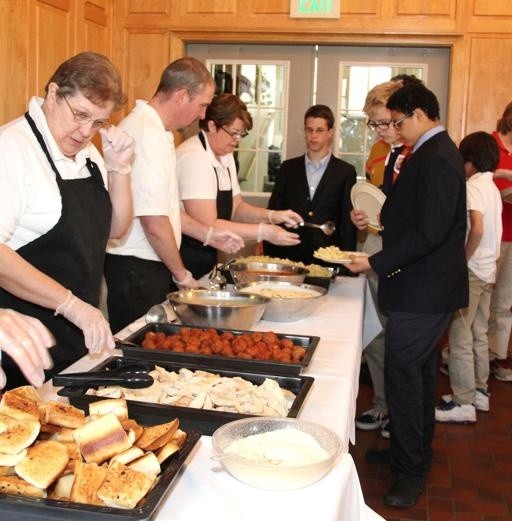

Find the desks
[[2, 251, 389, 520]]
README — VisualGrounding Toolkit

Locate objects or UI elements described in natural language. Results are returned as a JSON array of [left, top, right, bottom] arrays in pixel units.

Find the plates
[[501, 187, 511, 204], [313, 252, 370, 265], [350, 182, 387, 233]]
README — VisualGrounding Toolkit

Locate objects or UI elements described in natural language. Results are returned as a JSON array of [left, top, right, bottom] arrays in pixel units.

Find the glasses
[[394, 113, 413, 130], [61, 94, 112, 128], [220, 126, 249, 139], [366, 120, 391, 131]]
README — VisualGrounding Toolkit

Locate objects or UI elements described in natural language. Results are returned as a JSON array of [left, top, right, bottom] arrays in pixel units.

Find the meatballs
[[141, 326, 306, 363]]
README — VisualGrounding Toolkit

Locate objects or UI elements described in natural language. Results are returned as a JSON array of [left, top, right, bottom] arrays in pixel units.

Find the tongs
[[50, 361, 153, 393]]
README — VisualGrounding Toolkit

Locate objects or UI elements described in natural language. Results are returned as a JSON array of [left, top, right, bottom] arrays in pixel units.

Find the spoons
[[299, 222, 337, 237]]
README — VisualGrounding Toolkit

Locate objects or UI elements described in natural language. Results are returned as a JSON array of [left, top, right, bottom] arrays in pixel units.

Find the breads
[[85, 365, 296, 417], [0, 385, 187, 510]]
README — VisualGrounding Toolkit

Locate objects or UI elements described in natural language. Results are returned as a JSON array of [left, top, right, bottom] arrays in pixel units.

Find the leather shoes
[[367, 450, 391, 464], [384, 479, 424, 507]]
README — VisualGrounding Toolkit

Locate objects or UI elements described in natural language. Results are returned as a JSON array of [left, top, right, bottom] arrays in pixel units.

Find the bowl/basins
[[211, 416, 341, 495], [166, 260, 334, 332], [145, 305, 167, 324]]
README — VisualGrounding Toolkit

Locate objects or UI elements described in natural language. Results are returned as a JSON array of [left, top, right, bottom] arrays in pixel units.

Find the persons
[[348, 82, 404, 438], [261, 103, 360, 276], [438, 101, 511, 383], [0, 307, 56, 391], [434, 131, 504, 425], [176, 94, 305, 280], [104, 56, 245, 341], [0, 51, 136, 396], [342, 83, 469, 508], [390, 74, 422, 89]]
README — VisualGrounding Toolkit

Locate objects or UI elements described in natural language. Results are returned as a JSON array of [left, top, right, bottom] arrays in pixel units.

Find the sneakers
[[354, 409, 390, 439], [433, 362, 512, 423]]
